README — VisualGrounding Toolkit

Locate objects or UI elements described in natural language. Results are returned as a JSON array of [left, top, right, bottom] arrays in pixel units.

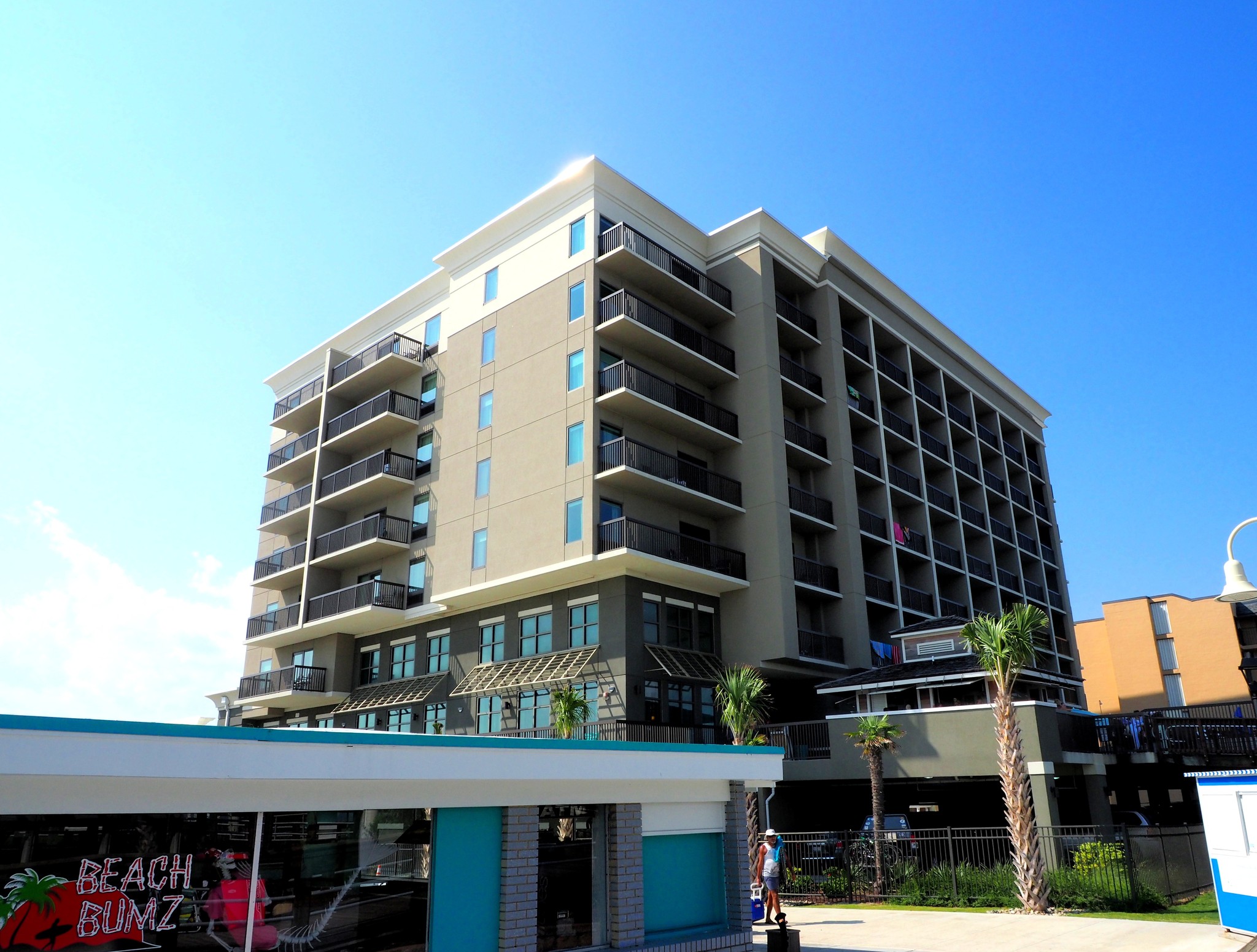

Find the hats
[[764, 829, 780, 841]]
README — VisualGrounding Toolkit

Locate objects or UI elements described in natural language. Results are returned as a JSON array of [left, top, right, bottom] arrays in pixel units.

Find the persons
[[1131, 710, 1147, 752], [1056, 703, 1071, 711], [884, 697, 982, 711], [756, 829, 796, 924]]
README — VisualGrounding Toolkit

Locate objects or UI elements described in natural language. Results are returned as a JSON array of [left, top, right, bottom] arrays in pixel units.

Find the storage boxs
[[750, 896, 764, 922]]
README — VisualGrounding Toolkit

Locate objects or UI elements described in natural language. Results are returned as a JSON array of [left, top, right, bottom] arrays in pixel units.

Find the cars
[[796, 833, 845, 866]]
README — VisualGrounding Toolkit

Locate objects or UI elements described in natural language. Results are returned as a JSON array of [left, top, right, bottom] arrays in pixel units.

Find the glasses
[[768, 834, 776, 838]]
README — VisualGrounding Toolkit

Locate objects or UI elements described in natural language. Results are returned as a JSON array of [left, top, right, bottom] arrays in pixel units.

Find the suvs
[[858, 814, 920, 856]]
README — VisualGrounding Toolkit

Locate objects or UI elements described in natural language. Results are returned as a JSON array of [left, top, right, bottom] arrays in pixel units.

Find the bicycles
[[842, 830, 904, 869]]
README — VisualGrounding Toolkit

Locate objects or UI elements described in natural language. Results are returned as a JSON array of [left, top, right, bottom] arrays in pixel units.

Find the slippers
[[784, 920, 788, 924], [765, 921, 776, 924]]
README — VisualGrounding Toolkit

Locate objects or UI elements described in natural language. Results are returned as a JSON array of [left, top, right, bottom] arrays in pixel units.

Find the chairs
[[285, 672, 314, 690], [374, 589, 396, 607], [148, 859, 200, 933], [403, 347, 420, 362]]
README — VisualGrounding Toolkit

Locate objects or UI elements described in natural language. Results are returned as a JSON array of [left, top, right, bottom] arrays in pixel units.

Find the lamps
[[504, 702, 510, 709], [376, 719, 381, 725], [412, 713, 418, 721], [458, 707, 463, 712], [342, 723, 345, 727], [608, 686, 614, 692]]
